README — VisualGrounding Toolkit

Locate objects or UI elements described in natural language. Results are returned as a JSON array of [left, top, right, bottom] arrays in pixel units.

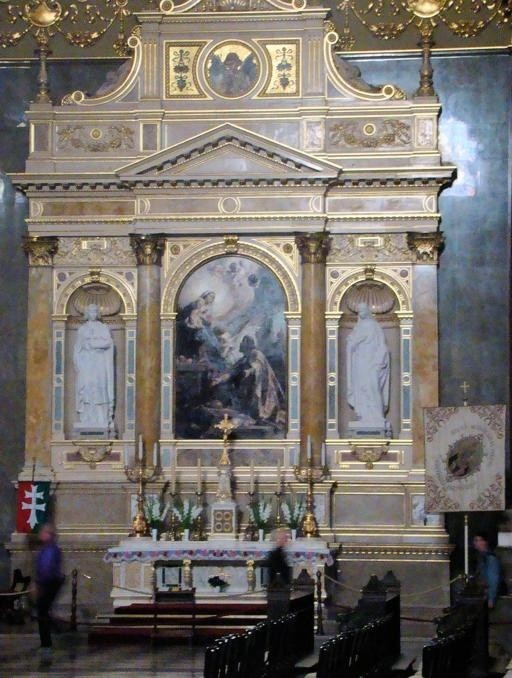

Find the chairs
[[306, 567, 418, 678], [203, 604, 333, 677], [414, 588, 499, 677]]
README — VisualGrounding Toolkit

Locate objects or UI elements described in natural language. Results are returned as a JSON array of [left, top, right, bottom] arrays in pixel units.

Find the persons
[[33, 524, 62, 655], [267, 530, 293, 616], [203, 50, 260, 95], [346, 303, 390, 429], [178, 292, 285, 424], [474, 535, 501, 610], [72, 303, 115, 430]]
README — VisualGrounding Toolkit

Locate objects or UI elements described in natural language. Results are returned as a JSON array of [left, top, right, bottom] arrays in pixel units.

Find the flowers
[[142, 493, 307, 543]]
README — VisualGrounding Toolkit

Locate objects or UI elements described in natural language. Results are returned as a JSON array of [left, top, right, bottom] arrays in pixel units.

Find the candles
[[120, 426, 336, 504]]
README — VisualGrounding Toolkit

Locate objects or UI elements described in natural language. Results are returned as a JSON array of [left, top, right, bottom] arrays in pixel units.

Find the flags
[[18, 481, 49, 533]]
